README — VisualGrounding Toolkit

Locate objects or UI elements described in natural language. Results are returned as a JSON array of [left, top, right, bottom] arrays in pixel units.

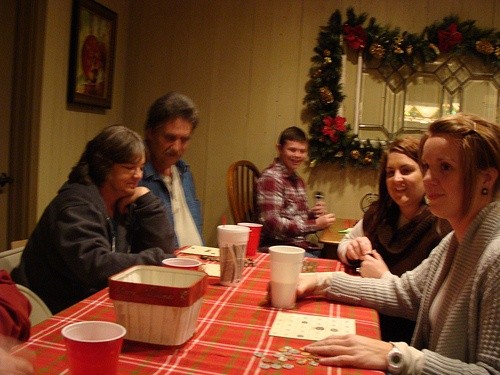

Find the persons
[[337, 134, 452, 343], [135, 91, 204, 252], [10, 124, 199, 315], [264, 112, 500, 375], [256, 126, 336, 257], [0, 268, 33, 375]]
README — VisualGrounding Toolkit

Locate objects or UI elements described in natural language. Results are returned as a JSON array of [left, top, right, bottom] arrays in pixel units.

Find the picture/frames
[[67, 0, 120, 110]]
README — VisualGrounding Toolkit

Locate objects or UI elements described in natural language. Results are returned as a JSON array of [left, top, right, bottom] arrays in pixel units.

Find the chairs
[[227, 160, 262, 225]]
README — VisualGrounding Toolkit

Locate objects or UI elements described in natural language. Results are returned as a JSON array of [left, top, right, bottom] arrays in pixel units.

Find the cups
[[61, 321, 126, 375], [162, 258, 201, 272], [237, 222, 262, 256], [268, 246, 305, 309], [218, 224, 250, 287]]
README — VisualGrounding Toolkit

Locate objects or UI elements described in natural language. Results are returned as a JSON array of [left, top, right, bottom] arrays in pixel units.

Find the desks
[[9, 244, 388, 375], [317, 214, 360, 244]]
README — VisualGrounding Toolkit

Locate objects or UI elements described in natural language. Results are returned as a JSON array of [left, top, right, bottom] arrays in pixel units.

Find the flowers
[[370, 42, 384, 60], [319, 88, 335, 106], [439, 23, 462, 53], [476, 37, 494, 55], [343, 25, 367, 51], [321, 114, 346, 143]]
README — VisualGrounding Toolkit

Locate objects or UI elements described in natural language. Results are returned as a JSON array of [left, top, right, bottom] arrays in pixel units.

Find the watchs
[[387, 343, 406, 375]]
